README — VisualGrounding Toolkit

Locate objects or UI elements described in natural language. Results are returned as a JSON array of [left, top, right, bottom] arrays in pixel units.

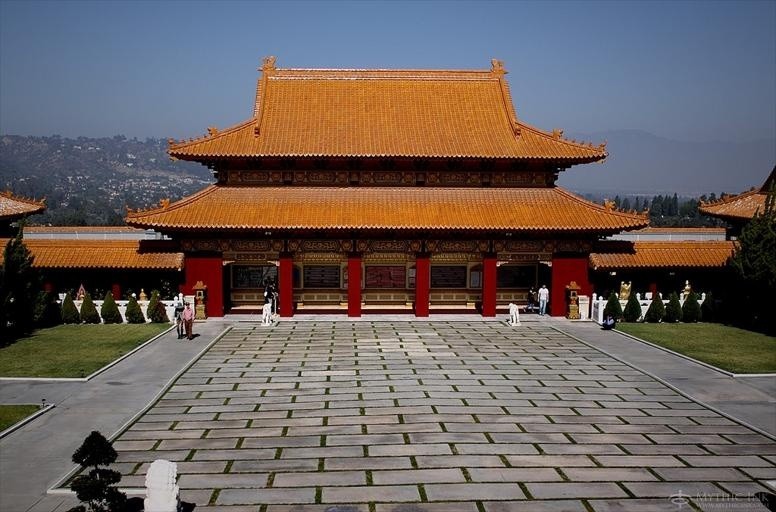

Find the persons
[[620, 280, 633, 301], [260, 277, 279, 327], [174, 303, 185, 339], [603, 313, 616, 329], [523, 285, 537, 313], [536, 284, 550, 316], [182, 302, 195, 339]]
